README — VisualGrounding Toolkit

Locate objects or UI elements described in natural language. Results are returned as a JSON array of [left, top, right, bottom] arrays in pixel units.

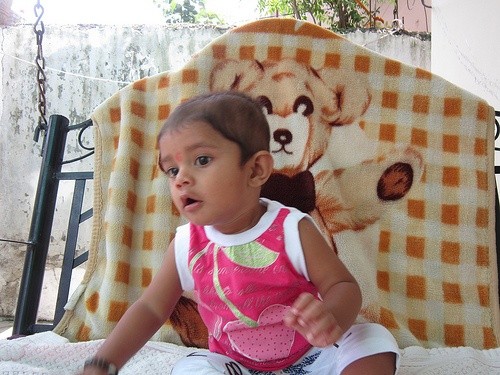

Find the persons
[[81, 91, 401, 375]]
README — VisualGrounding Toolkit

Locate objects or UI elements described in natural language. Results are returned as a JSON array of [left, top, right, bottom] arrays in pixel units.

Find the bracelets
[[83, 356, 118, 375]]
